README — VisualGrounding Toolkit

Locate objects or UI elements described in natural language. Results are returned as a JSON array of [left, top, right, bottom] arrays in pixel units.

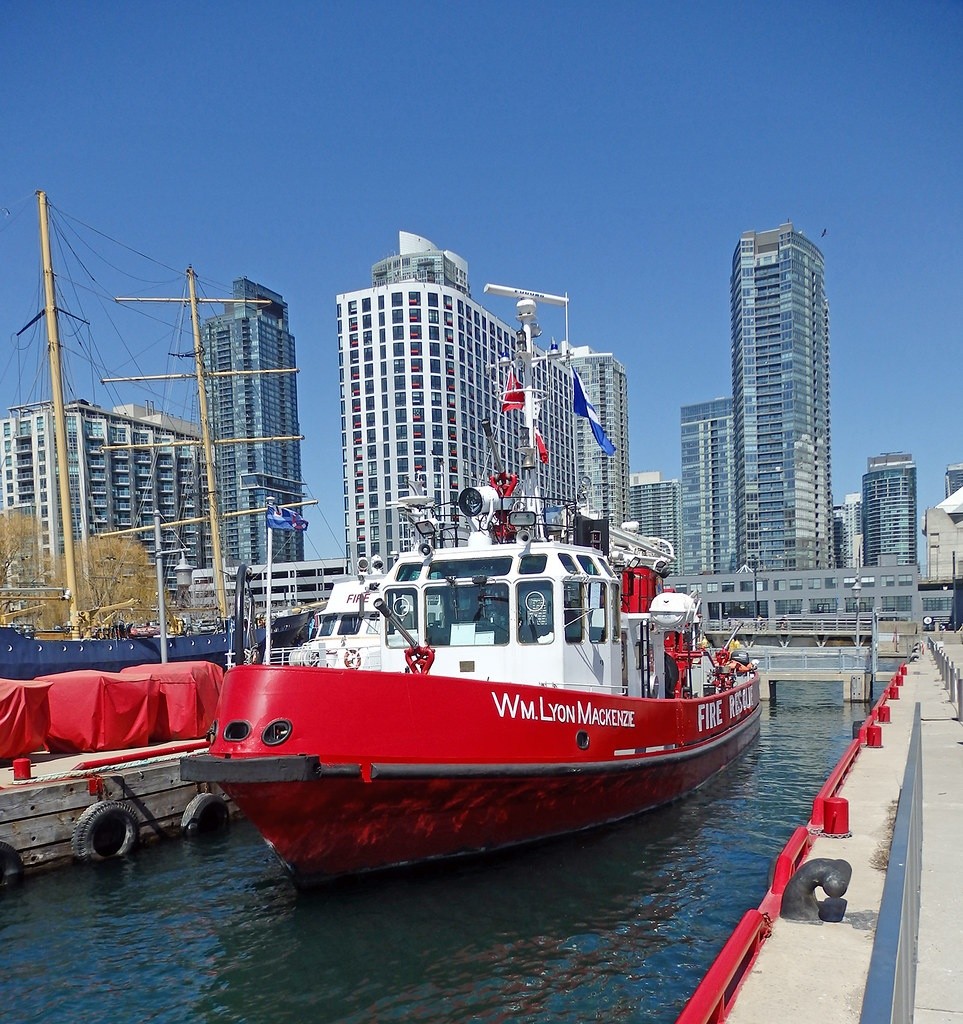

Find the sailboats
[[0, 189, 328, 682]]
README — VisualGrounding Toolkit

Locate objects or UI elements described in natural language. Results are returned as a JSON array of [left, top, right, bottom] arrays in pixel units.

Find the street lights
[[751, 554, 758, 631], [851, 576, 862, 668]]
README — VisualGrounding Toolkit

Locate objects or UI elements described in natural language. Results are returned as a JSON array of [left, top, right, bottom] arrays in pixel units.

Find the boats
[[179, 284, 760, 899]]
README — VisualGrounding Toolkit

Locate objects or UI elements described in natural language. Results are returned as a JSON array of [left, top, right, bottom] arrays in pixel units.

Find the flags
[[266, 505, 309, 532], [572, 366, 616, 457], [534, 424, 549, 464], [502, 370, 525, 412]]
